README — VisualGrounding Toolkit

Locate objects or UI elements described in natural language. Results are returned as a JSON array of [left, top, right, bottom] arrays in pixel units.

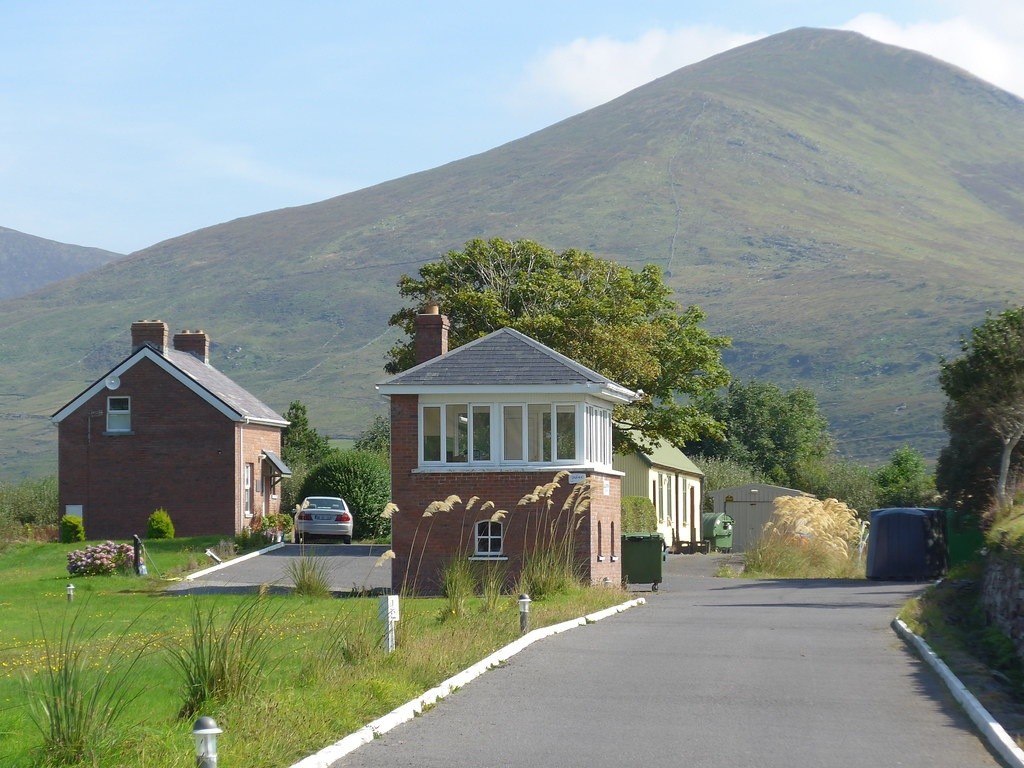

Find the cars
[[294, 496, 354, 544]]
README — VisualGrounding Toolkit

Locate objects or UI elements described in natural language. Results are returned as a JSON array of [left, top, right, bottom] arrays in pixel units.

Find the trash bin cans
[[620, 531, 664, 591], [701, 513, 735, 554]]
